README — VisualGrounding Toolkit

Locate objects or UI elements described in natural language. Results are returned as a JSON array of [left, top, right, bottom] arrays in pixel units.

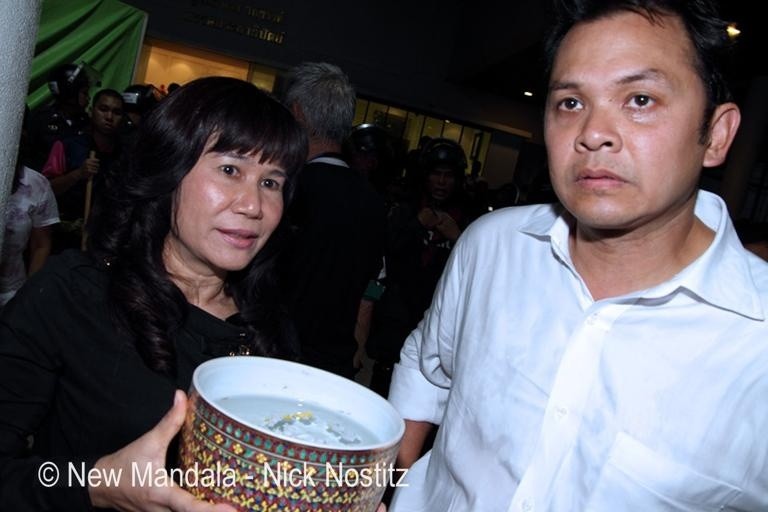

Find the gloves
[[71, 157, 101, 182]]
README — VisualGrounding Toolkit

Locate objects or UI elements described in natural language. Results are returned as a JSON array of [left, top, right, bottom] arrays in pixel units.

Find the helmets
[[417, 137, 467, 173], [49, 60, 104, 103], [122, 84, 161, 118]]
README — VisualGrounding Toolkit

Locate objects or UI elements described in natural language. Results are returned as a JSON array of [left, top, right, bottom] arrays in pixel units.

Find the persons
[[388, 3, 767, 512], [3, 62, 504, 396], [1, 75, 388, 512]]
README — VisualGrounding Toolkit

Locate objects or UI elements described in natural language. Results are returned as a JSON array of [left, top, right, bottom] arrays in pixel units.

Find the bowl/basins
[[177, 354, 405, 512]]
